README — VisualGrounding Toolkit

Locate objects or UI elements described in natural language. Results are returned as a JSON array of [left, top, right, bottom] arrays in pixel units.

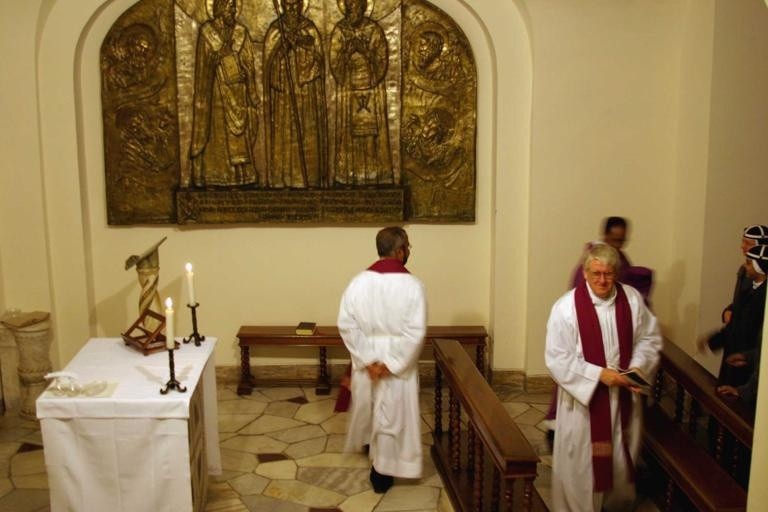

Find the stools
[[9, 319, 53, 422]]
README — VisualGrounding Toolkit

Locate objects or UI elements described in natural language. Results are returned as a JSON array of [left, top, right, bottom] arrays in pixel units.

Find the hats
[[742, 224, 768, 246], [745, 245, 768, 275]]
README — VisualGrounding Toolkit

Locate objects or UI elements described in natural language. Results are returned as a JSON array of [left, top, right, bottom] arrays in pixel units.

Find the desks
[[236, 326, 488, 396], [35, 336, 221, 512]]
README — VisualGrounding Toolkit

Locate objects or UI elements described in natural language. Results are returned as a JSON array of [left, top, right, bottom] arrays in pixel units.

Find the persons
[[188, 0, 262, 189], [336, 226, 428, 495], [540, 215, 658, 458], [403, 104, 470, 216], [326, 0, 395, 188], [405, 30, 445, 85], [109, 103, 169, 163], [257, 0, 330, 191], [543, 244, 663, 512], [694, 245, 768, 412], [108, 26, 157, 77], [714, 221, 768, 391]]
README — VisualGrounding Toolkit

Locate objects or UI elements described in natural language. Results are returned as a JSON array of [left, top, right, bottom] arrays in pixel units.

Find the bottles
[[53, 375, 107, 398]]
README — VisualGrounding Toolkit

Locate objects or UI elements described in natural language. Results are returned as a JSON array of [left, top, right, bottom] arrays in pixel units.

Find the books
[[611, 365, 654, 390], [295, 321, 317, 336]]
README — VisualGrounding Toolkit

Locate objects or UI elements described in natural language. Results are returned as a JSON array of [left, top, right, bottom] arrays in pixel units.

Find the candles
[[186, 263, 196, 307], [165, 296, 175, 349]]
